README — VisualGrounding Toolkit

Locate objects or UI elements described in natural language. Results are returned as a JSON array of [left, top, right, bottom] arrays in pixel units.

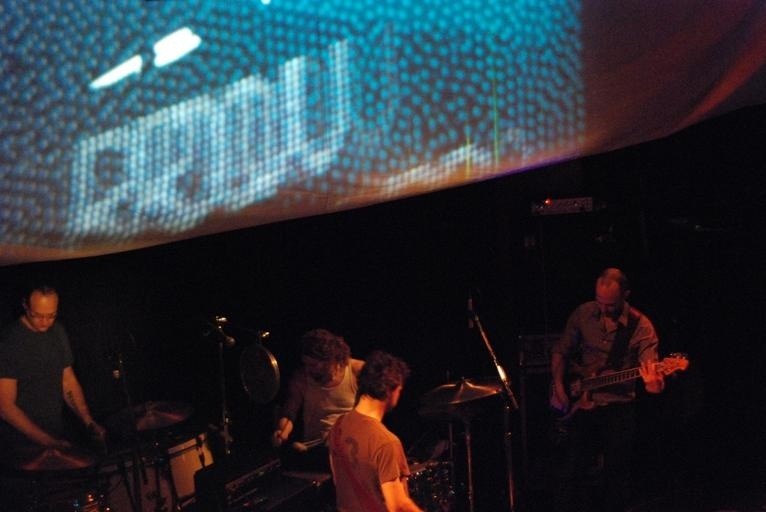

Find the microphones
[[206, 322, 236, 347], [111, 349, 120, 379], [467, 296, 475, 329]]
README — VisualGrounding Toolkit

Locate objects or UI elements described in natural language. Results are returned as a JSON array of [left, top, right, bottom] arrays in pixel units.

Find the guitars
[[548, 352, 690, 415]]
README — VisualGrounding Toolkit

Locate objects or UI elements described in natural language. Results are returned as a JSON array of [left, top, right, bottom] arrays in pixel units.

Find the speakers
[[519, 366, 558, 486]]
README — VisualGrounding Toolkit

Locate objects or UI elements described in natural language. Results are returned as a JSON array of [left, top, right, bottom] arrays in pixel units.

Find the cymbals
[[421, 379, 503, 406], [104, 399, 193, 432], [7, 445, 97, 473], [239, 345, 280, 402]]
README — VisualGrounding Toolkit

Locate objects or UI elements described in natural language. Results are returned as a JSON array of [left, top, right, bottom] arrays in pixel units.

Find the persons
[[271, 327, 365, 473], [549, 267, 668, 510], [0, 282, 108, 450], [328, 350, 428, 511]]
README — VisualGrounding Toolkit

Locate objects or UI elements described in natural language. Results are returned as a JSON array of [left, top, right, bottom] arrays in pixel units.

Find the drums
[[404, 459, 457, 511], [285, 469, 336, 511], [94, 432, 214, 508]]
